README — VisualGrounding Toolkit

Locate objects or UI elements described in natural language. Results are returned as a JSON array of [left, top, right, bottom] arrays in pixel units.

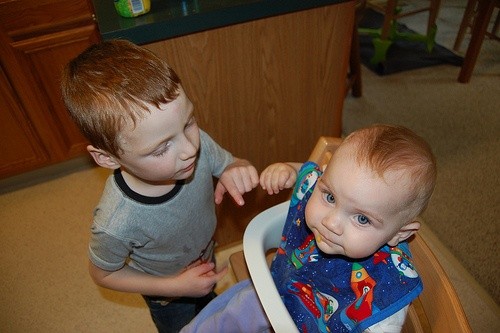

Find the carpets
[[358, 7, 464, 76]]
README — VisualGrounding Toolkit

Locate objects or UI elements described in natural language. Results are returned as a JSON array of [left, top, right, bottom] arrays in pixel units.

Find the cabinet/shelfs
[[0, 1, 351, 260]]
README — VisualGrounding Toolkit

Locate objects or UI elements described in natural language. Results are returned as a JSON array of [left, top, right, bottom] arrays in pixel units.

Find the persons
[[59, 38, 260, 333], [178, 122, 438, 333]]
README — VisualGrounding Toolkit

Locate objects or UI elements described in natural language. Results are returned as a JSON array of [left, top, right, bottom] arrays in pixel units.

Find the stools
[[455, 0, 500, 82]]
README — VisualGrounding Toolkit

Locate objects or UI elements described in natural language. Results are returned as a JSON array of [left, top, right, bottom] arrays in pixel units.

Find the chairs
[[229, 137, 473, 333]]
